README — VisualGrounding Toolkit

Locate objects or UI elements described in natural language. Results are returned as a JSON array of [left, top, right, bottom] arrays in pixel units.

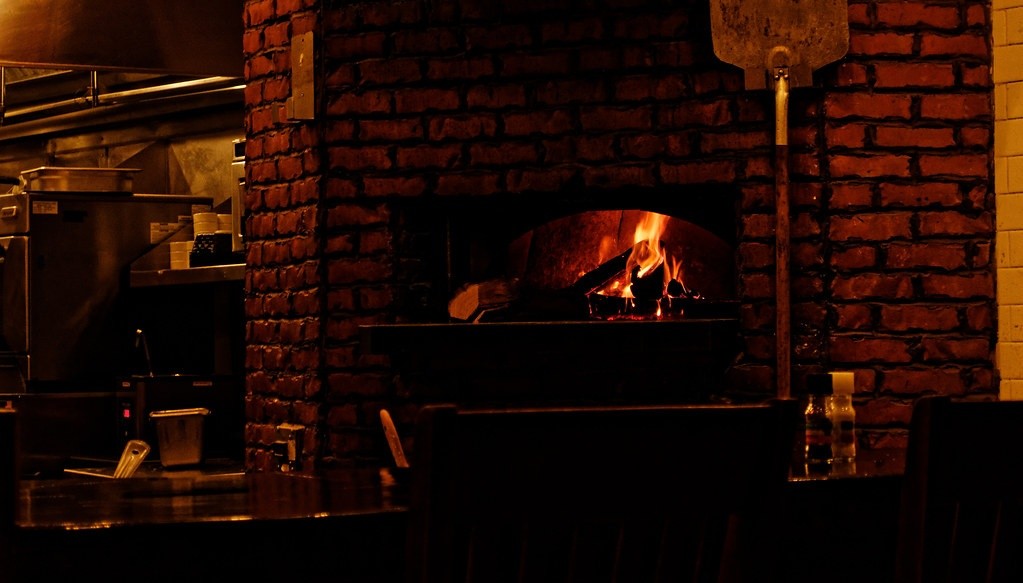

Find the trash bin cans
[[132, 373, 199, 466], [148, 408, 210, 469]]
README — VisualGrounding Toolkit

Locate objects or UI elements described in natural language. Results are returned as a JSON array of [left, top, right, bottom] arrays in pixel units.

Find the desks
[[133, 259, 249, 463], [13, 472, 906, 583]]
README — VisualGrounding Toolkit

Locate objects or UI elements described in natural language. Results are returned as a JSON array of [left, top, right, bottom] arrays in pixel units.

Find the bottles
[[804, 372, 856, 480]]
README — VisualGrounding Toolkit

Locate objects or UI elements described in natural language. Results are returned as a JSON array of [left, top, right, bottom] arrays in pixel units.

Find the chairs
[[416, 399, 803, 583], [380, 405, 421, 468], [903, 396, 1022, 583]]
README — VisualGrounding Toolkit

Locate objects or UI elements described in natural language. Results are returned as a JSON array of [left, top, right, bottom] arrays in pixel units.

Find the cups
[[170, 213, 232, 269]]
